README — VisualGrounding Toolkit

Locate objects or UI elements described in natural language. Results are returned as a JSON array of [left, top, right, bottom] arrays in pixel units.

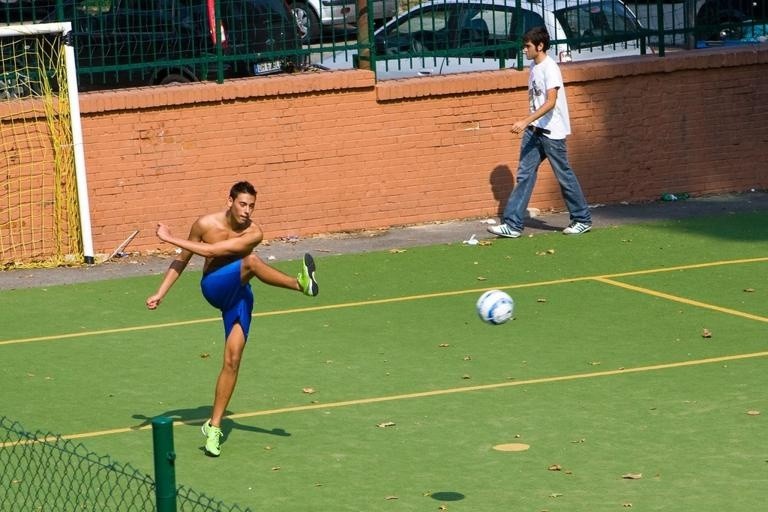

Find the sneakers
[[562, 221, 593, 236], [296, 252, 319, 297], [200, 416, 224, 457], [484, 223, 521, 239]]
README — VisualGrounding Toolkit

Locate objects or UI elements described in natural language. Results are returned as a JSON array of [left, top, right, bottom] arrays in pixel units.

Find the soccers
[[475, 289, 514, 324]]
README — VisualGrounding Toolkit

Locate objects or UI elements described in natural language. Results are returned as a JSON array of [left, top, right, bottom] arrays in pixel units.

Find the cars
[[310, 0, 657, 82], [0, 0, 307, 100], [284, 0, 400, 46]]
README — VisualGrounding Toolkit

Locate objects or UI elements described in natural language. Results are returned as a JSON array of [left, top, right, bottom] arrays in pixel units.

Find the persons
[[144, 179, 321, 458], [485, 25, 594, 240]]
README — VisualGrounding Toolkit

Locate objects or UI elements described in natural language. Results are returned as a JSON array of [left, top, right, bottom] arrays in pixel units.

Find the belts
[[527, 125, 550, 135]]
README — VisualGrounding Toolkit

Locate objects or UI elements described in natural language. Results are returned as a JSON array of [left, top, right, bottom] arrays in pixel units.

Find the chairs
[[459, 12, 609, 48]]
